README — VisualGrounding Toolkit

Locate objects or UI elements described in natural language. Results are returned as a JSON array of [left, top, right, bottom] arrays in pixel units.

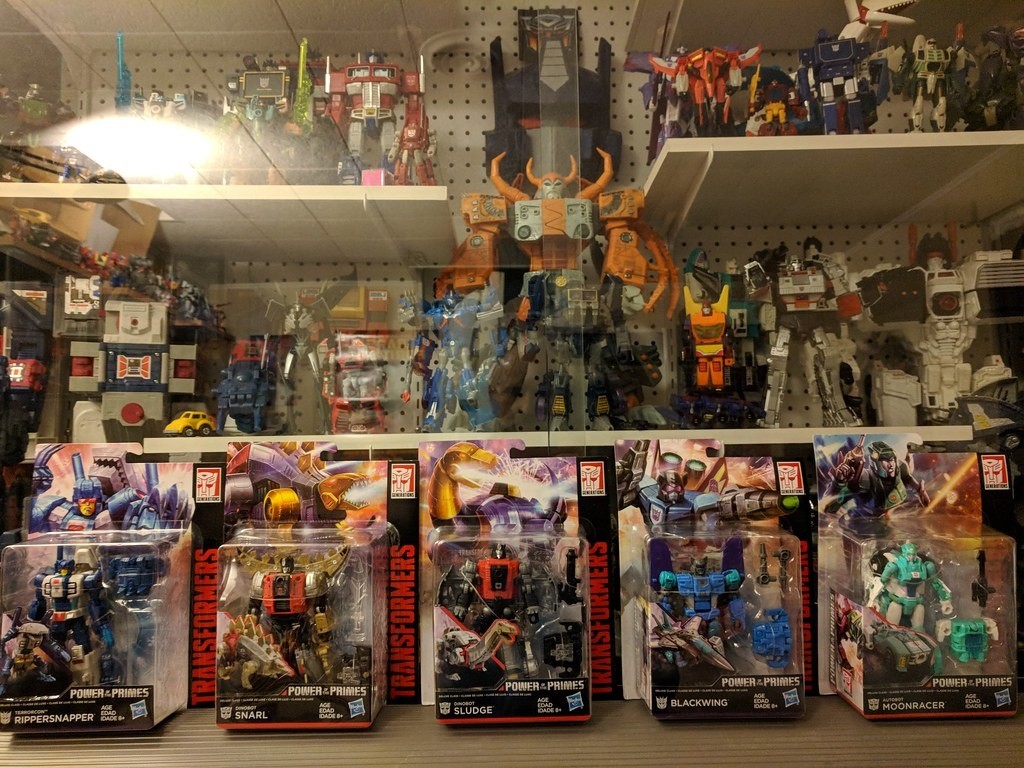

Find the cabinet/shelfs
[[0, 0, 1024, 768]]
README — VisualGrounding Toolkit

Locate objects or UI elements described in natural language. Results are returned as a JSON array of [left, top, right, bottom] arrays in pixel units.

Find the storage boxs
[[0, 432, 1024, 734]]
